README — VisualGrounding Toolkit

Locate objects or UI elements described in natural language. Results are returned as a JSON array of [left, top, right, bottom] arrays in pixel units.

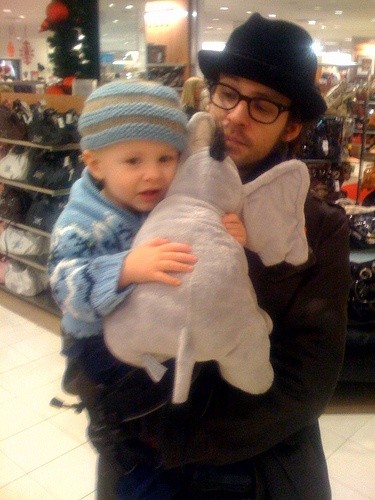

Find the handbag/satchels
[[146, 63, 187, 86], [337, 199, 375, 249], [348, 259, 375, 320], [0, 98, 85, 297]]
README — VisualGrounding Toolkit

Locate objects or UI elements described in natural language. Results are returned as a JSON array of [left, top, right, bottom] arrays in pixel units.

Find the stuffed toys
[[103, 111, 309, 404]]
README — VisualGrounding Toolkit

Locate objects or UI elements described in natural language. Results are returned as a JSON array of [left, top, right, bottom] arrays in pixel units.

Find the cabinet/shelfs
[[0, 90, 87, 337], [296, 57, 375, 394]]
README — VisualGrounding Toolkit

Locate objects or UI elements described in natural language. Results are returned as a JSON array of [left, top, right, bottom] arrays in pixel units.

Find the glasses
[[208, 82, 293, 124]]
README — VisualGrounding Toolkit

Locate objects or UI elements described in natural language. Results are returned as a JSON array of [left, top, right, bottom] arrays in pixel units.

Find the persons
[[84, 13, 347, 498], [47, 79, 247, 394], [179, 77, 209, 119]]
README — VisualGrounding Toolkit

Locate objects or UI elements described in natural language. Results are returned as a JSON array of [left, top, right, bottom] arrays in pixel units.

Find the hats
[[78, 79, 189, 152], [199, 12, 328, 123]]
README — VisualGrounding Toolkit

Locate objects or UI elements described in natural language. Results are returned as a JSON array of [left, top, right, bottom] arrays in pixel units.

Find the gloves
[[113, 440, 167, 500]]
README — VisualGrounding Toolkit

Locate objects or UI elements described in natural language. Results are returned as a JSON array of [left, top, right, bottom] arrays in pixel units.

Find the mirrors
[[97, 1, 197, 92]]
